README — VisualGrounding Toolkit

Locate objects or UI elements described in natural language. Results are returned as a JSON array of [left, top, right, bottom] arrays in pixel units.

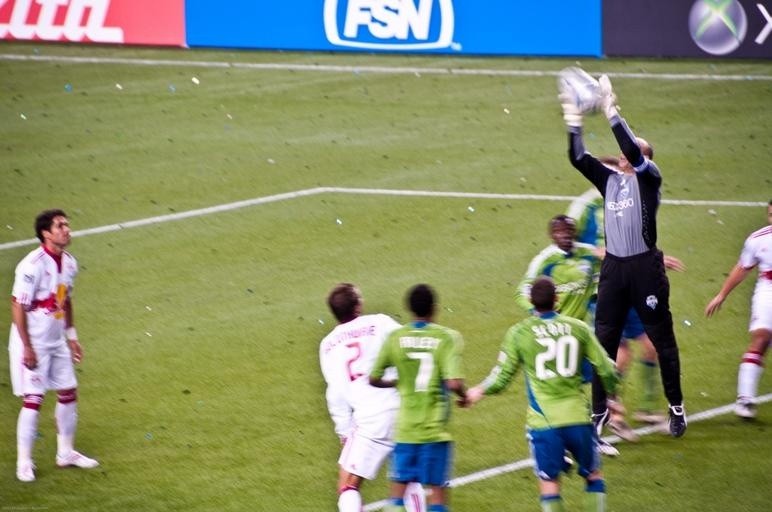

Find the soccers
[[559, 67, 602, 114]]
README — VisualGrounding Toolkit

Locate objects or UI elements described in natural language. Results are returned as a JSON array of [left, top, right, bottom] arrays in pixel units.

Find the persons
[[464, 273, 622, 512], [317, 280, 428, 512], [510, 212, 622, 471], [369, 281, 471, 511], [555, 92, 686, 438], [702, 199, 772, 421], [563, 152, 686, 443], [7, 208, 99, 484]]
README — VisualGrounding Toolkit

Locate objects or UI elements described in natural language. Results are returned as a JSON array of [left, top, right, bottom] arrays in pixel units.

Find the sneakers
[[54, 449, 100, 469], [667, 402, 689, 440], [595, 436, 621, 458], [15, 457, 37, 483], [604, 410, 666, 444], [733, 393, 759, 419]]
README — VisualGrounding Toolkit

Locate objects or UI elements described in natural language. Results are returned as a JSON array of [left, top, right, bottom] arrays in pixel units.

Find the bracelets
[[61, 326, 79, 343]]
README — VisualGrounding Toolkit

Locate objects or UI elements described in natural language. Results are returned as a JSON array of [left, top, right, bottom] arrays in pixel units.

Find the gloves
[[557, 87, 583, 128], [597, 71, 620, 121]]
